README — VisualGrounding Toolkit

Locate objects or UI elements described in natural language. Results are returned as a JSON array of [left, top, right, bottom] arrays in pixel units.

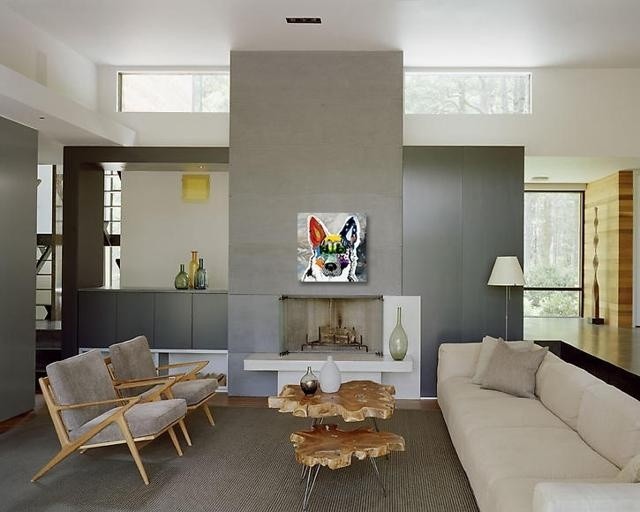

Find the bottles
[[203, 269, 208, 288], [194, 258, 206, 289], [174, 265, 189, 289]]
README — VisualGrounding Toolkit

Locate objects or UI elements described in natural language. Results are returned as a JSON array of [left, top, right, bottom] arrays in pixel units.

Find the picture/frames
[[297, 213, 369, 284]]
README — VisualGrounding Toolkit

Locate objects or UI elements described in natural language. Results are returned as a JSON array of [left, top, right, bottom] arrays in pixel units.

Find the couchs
[[437, 338, 638, 511]]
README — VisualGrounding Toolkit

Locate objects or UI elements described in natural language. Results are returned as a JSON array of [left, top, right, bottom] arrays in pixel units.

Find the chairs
[[30, 349, 186, 488], [105, 335, 216, 443]]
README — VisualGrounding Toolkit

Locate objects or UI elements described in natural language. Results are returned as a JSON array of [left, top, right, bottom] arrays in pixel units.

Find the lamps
[[487, 256, 526, 341]]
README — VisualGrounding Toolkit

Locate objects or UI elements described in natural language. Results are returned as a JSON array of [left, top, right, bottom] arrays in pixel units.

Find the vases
[[174, 264, 190, 290], [317, 355, 342, 394], [301, 367, 320, 396], [389, 305, 407, 361], [193, 258, 208, 290]]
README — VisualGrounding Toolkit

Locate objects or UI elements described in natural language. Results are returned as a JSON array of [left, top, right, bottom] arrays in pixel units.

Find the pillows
[[480, 336, 549, 399], [471, 334, 535, 386]]
